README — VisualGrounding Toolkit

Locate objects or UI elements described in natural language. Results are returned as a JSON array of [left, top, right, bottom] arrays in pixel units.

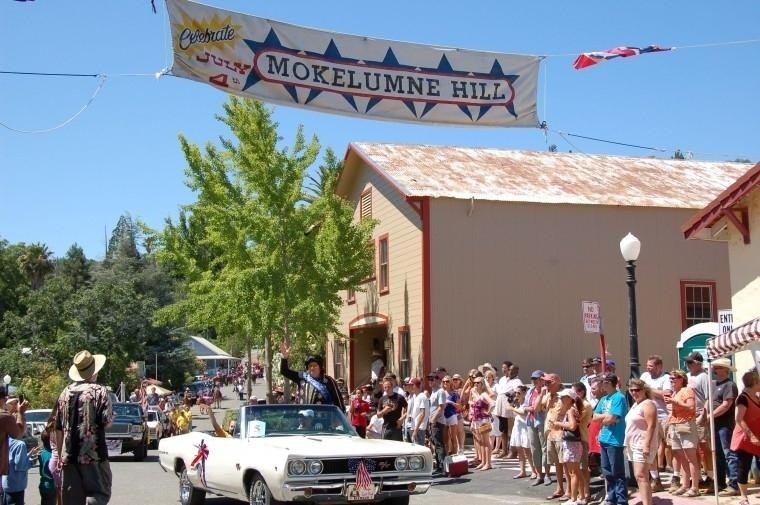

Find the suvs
[[104, 403, 148, 459], [25, 408, 53, 435]]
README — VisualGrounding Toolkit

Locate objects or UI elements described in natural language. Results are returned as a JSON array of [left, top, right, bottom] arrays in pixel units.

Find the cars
[[22, 422, 47, 447], [181, 380, 213, 400], [147, 409, 163, 448]]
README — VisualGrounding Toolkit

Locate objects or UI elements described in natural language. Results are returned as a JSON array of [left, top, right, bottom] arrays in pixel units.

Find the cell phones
[[19, 394, 25, 405], [548, 419, 553, 425]]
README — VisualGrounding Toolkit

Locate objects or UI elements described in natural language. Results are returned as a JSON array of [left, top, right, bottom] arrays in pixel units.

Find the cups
[[663, 393, 671, 398]]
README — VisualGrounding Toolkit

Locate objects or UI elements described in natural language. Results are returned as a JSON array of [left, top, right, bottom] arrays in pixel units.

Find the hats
[[530, 356, 618, 402], [682, 351, 703, 363], [304, 355, 323, 370], [478, 363, 496, 375], [68, 350, 106, 382], [710, 357, 737, 372], [383, 367, 446, 388]]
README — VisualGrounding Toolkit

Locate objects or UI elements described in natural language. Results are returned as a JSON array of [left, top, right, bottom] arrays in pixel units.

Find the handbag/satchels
[[561, 412, 581, 441], [479, 422, 492, 434]]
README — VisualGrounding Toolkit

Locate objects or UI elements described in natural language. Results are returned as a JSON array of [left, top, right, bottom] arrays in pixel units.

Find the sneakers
[[512, 473, 552, 486], [492, 450, 517, 459], [468, 458, 492, 471], [546, 492, 591, 505], [651, 476, 758, 497]]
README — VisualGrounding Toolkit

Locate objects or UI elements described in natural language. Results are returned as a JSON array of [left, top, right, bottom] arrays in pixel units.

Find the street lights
[[3, 373, 11, 396], [620, 230, 643, 383]]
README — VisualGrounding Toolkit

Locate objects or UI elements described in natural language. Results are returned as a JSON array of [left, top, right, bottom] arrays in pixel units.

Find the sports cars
[[158, 404, 434, 503]]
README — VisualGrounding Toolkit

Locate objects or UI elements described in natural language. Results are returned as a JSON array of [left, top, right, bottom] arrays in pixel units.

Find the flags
[[571, 44, 671, 71]]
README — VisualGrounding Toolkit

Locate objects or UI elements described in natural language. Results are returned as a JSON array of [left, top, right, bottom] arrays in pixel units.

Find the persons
[[105, 385, 119, 403], [128, 362, 262, 438], [298, 408, 317, 430], [278, 342, 347, 431], [0, 349, 115, 505], [347, 350, 760, 505]]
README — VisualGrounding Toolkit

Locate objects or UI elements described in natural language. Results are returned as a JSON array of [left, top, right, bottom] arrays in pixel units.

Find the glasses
[[452, 375, 480, 386], [628, 388, 643, 392], [687, 361, 695, 364]]
